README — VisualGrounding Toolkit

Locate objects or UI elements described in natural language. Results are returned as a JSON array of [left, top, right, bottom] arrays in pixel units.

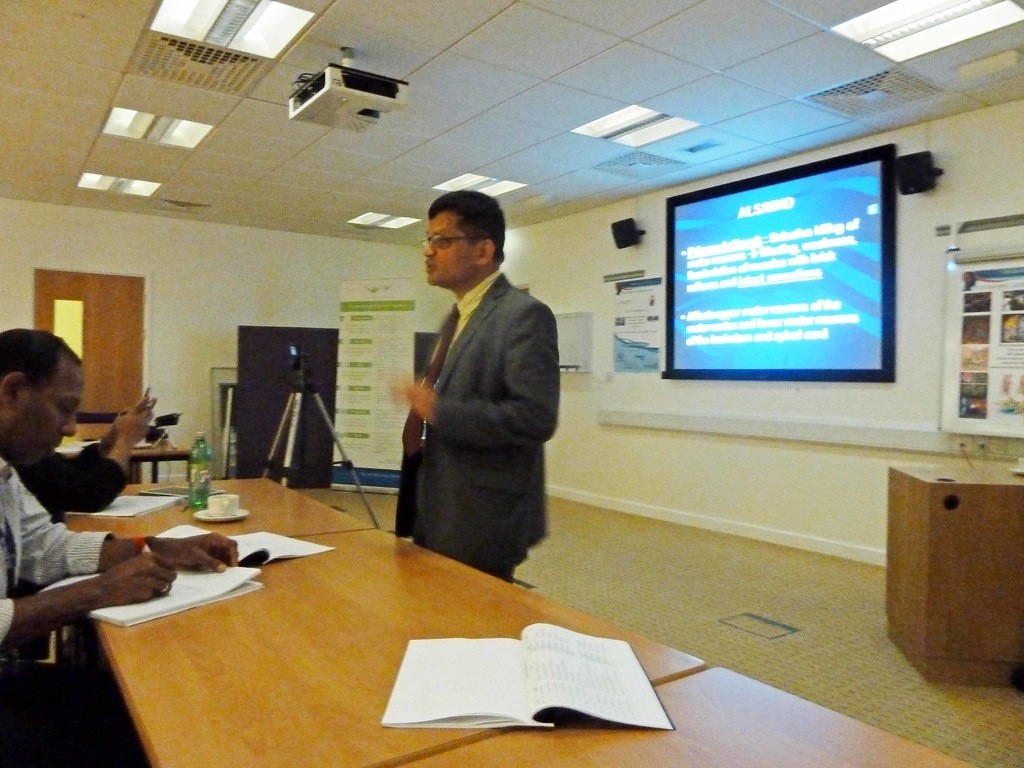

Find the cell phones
[[144, 383, 152, 398]]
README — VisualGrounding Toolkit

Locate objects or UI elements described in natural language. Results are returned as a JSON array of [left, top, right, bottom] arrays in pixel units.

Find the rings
[[161, 585, 169, 593]]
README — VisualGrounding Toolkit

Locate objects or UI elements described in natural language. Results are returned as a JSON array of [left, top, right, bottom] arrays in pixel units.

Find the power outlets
[[975, 436, 990, 451], [955, 436, 972, 452]]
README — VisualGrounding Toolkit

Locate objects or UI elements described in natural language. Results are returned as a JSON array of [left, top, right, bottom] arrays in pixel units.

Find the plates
[[194, 509, 249, 522]]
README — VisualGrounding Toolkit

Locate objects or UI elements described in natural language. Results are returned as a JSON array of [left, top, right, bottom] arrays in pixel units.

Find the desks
[[55, 476, 976, 768], [130, 444, 190, 484]]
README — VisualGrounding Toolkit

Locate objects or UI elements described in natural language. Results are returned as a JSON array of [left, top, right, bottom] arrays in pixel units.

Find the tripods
[[260, 359, 380, 531]]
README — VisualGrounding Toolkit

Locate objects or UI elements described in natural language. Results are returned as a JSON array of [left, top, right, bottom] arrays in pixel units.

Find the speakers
[[610, 217, 640, 249], [893, 150, 936, 196]]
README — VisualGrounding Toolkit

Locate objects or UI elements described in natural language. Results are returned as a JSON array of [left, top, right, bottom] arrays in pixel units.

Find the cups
[[207, 494, 240, 517]]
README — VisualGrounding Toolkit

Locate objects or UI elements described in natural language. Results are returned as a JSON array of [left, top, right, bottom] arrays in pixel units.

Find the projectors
[[286, 61, 411, 135]]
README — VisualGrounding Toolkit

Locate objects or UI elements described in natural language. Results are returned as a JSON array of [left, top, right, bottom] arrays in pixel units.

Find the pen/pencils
[[133, 535, 152, 554]]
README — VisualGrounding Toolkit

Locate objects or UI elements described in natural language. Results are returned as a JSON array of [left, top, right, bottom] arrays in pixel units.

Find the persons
[[0, 328, 241, 768], [393, 191, 561, 584]]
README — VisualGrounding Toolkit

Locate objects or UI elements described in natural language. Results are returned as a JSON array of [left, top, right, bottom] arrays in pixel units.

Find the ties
[[402, 303, 460, 457]]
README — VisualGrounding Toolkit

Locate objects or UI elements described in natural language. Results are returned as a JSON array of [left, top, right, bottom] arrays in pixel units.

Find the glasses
[[421, 232, 499, 250]]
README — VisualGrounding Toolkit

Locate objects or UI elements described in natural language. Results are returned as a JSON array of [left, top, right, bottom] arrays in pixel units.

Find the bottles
[[189, 432, 213, 508]]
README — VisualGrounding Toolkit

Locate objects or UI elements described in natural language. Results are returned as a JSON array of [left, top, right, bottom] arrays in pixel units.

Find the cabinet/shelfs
[[884, 462, 1024, 688]]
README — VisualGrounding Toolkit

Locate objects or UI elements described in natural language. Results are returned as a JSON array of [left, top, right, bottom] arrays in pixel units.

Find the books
[[38, 565, 263, 627], [381, 623, 675, 730], [65, 495, 187, 518], [156, 524, 336, 564]]
